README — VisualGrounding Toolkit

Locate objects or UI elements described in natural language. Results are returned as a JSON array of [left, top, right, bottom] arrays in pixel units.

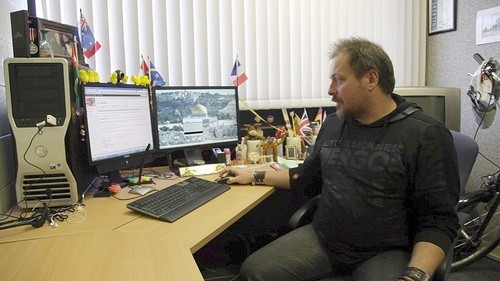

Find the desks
[[0, 151, 304, 281]]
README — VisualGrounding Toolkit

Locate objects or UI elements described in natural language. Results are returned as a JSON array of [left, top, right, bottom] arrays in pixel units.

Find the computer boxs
[[3, 57, 97, 208]]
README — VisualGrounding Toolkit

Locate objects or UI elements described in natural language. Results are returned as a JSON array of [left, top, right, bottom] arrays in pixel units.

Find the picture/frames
[[428, 0, 457, 36], [476, 6, 500, 45]]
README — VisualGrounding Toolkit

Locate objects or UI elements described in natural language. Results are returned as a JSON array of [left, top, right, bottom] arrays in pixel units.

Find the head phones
[[0, 210, 45, 229]]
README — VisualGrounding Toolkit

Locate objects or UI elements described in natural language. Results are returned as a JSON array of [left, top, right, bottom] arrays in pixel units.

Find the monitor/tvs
[[81, 84, 156, 186], [153, 84, 240, 176], [393, 86, 462, 132]]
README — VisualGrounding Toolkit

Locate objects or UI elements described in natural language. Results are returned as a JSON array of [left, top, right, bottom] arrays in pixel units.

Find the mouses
[[216, 176, 235, 183]]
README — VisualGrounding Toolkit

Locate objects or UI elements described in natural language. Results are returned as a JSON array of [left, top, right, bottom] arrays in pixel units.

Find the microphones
[[40, 188, 52, 216]]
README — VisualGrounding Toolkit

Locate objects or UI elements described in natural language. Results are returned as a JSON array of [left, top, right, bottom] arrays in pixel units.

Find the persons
[[218, 35, 461, 281]]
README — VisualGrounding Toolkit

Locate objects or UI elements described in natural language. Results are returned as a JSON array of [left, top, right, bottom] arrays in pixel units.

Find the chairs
[[290, 130, 480, 281]]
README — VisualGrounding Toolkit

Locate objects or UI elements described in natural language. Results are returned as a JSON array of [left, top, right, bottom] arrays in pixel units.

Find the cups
[[247, 140, 262, 164]]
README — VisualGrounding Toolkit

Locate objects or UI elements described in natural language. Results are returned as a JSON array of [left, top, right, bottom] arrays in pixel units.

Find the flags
[[314, 108, 322, 121], [149, 60, 166, 87], [230, 59, 248, 87], [296, 108, 313, 146], [79, 7, 102, 58], [140, 60, 149, 76]]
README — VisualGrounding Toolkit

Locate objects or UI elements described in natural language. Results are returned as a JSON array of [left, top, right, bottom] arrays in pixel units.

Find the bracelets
[[253, 171, 266, 185], [398, 267, 432, 281]]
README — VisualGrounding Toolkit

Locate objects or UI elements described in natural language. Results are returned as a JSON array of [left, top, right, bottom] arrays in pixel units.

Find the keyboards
[[125, 176, 230, 223]]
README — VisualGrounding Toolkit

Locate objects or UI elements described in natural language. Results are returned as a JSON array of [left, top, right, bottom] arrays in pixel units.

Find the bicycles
[[444, 52, 499, 271]]
